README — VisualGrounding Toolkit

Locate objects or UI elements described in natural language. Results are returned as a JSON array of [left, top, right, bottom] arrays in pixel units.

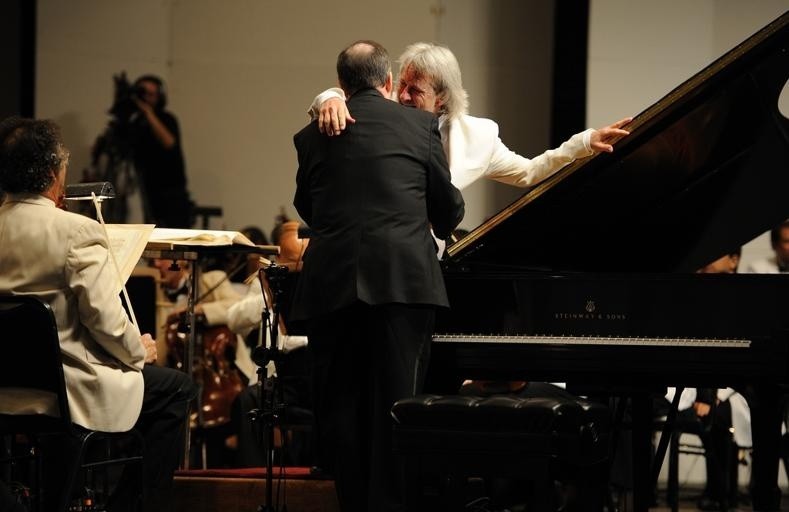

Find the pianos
[[141, 227, 280, 284], [431, 13, 785, 510]]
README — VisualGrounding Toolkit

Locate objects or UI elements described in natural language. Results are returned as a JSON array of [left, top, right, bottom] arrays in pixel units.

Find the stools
[[389, 392, 594, 512]]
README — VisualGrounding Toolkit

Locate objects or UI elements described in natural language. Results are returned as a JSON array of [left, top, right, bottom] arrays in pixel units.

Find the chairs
[[0, 289, 149, 512]]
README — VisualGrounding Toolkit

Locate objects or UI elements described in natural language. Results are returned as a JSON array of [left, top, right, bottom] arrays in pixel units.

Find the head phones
[[135, 75, 165, 110]]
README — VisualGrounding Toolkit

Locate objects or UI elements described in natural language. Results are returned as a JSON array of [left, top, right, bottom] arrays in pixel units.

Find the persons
[[0, 111, 201, 510], [238, 227, 273, 263], [303, 41, 634, 272], [732, 218, 789, 274], [217, 220, 312, 470], [111, 75, 197, 229], [289, 39, 466, 510], [145, 248, 265, 447], [654, 244, 742, 512]]
